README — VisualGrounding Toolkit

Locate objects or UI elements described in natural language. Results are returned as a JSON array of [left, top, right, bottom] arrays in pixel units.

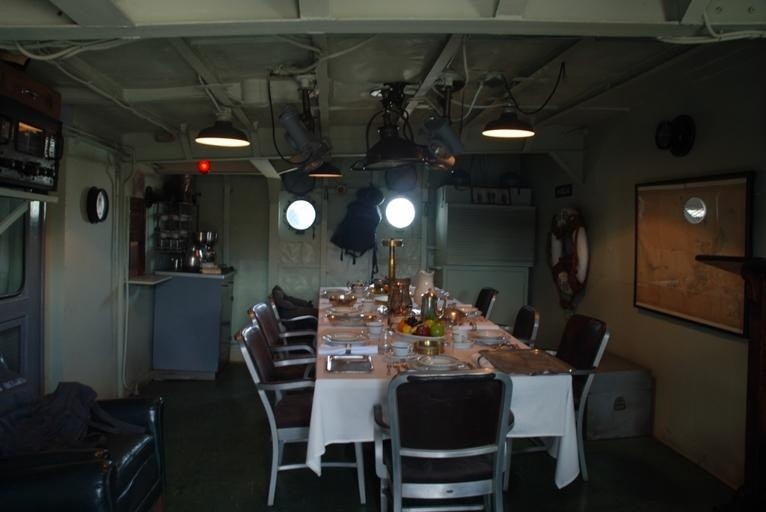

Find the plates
[[320, 285, 512, 372]]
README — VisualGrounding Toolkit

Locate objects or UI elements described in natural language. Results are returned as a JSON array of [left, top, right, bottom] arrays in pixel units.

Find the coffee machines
[[189, 230, 219, 267]]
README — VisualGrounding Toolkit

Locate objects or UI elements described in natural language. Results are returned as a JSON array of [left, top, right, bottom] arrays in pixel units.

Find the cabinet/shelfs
[[152, 269, 234, 381]]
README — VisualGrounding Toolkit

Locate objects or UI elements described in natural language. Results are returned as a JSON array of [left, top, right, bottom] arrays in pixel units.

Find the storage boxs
[[584, 352, 654, 442]]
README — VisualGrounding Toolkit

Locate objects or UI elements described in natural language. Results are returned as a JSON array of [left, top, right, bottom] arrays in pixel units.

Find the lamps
[[481, 95, 535, 138], [346, 88, 436, 171], [426, 87, 473, 192], [193, 105, 251, 147], [329, 172, 385, 259], [383, 166, 419, 229], [278, 169, 319, 237], [277, 88, 341, 179]]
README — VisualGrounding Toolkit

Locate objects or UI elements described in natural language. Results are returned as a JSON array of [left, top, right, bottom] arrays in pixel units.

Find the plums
[[415, 325, 430, 337]]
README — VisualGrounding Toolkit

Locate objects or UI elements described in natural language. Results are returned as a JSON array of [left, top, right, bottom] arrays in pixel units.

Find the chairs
[[235, 323, 368, 507], [246, 301, 316, 380], [371, 369, 515, 511], [510, 314, 611, 481], [268, 284, 318, 354], [498, 306, 540, 349], [474, 285, 500, 320], [0, 392, 166, 512]]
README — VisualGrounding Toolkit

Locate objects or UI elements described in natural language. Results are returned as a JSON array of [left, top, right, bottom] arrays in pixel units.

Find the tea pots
[[390, 268, 447, 323]]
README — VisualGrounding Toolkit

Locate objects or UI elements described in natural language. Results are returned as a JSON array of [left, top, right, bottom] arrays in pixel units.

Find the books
[[199, 262, 227, 269], [200, 267, 227, 275]]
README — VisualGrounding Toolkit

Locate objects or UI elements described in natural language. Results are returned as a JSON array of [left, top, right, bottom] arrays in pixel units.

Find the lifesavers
[[550, 207, 589, 294]]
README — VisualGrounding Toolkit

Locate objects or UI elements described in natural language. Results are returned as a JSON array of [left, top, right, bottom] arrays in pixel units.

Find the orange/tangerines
[[430, 325, 444, 337]]
